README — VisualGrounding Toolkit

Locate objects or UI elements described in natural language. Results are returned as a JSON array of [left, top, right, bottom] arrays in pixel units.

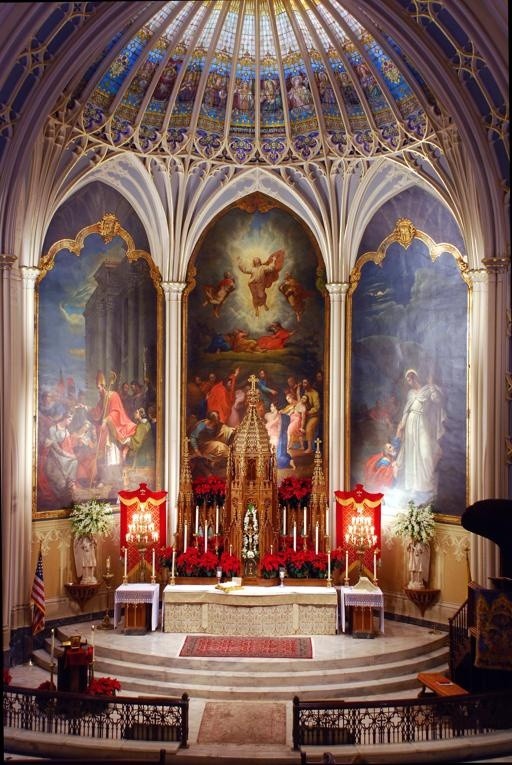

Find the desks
[[114, 583, 160, 635], [417, 673, 469, 699], [335, 585, 384, 639]]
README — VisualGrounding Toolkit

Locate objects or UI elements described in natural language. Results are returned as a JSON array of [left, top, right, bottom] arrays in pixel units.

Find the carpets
[[179, 636, 312, 659], [198, 701, 286, 745]]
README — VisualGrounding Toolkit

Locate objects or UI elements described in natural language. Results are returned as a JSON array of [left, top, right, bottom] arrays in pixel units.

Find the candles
[[283, 507, 286, 535], [196, 505, 198, 534], [106, 559, 110, 568], [91, 625, 95, 660], [374, 551, 376, 579], [316, 521, 318, 555], [328, 551, 330, 579], [346, 550, 348, 577], [172, 552, 175, 577], [184, 520, 187, 553], [51, 629, 54, 659], [152, 552, 155, 576], [205, 520, 208, 553], [304, 506, 306, 535], [124, 549, 127, 575], [293, 521, 296, 552], [216, 505, 219, 534]]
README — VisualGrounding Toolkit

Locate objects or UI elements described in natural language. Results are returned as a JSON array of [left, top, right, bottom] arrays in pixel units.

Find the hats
[[96, 370, 105, 385]]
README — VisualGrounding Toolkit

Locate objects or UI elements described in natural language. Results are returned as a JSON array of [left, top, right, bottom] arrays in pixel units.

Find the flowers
[[257, 536, 328, 580], [83, 677, 122, 696], [192, 476, 226, 507], [175, 536, 239, 577], [390, 498, 438, 547], [37, 680, 56, 691], [330, 551, 343, 573], [278, 474, 311, 510], [68, 495, 114, 540]]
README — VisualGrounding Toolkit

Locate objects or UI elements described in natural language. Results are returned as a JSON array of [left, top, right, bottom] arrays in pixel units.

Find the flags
[[28, 550, 47, 635]]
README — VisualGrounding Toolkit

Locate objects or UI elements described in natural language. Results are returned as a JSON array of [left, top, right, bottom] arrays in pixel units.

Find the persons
[[33, 368, 156, 492], [397, 369, 446, 496], [186, 368, 320, 463], [202, 256, 306, 353], [360, 430, 401, 487], [136, 60, 381, 110], [368, 398, 394, 430]]
